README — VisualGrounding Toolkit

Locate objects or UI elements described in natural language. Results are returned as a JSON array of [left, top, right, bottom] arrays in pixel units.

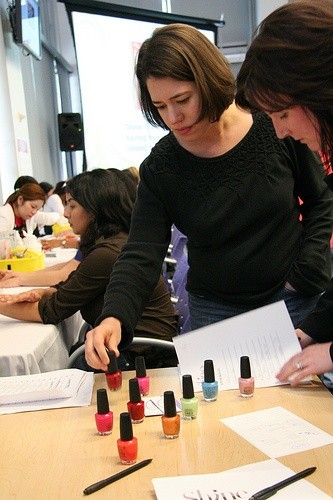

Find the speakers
[[59, 113, 84, 151]]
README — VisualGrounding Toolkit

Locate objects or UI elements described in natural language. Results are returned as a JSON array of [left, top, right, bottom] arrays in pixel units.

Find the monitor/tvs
[[12, 0, 42, 59]]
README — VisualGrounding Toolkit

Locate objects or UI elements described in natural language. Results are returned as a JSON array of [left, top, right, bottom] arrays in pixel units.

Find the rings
[[296, 362, 303, 370]]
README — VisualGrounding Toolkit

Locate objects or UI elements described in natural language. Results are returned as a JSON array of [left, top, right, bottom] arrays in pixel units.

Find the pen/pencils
[[82, 458, 153, 495], [250, 466, 318, 500]]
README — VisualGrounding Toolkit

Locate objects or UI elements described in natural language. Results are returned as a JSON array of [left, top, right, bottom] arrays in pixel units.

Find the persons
[[84, 22, 333, 374], [0, 168, 178, 373], [231, 0, 332, 391], [0, 165, 141, 260]]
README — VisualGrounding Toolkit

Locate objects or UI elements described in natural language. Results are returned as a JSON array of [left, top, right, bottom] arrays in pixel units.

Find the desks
[[0, 247, 86, 378], [0, 367, 333, 499]]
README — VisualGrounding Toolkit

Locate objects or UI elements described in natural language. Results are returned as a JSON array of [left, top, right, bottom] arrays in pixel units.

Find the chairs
[[63, 226, 191, 371]]
